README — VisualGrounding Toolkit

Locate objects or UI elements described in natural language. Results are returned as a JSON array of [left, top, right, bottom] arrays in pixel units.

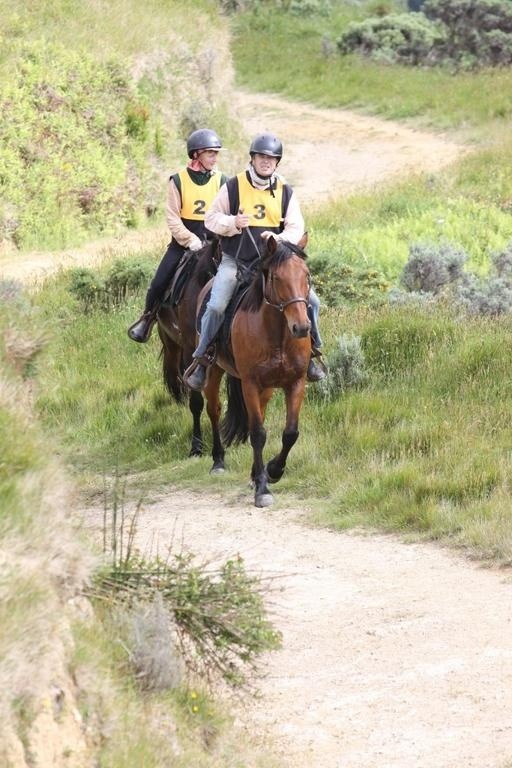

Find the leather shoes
[[186, 360, 208, 392], [126, 309, 160, 344], [305, 358, 327, 381]]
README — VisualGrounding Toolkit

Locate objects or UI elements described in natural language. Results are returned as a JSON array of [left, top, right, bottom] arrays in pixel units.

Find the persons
[[130, 127, 230, 344], [183, 132, 326, 395]]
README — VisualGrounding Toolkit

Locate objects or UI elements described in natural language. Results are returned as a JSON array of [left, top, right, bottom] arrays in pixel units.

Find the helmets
[[186, 128, 229, 158], [249, 133, 285, 158]]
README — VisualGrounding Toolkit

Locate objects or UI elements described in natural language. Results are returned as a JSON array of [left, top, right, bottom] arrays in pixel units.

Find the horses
[[194, 230, 314, 508], [158, 236, 221, 457]]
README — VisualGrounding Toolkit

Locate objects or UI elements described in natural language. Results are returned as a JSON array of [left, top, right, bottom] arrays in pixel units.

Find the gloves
[[187, 236, 203, 252]]
